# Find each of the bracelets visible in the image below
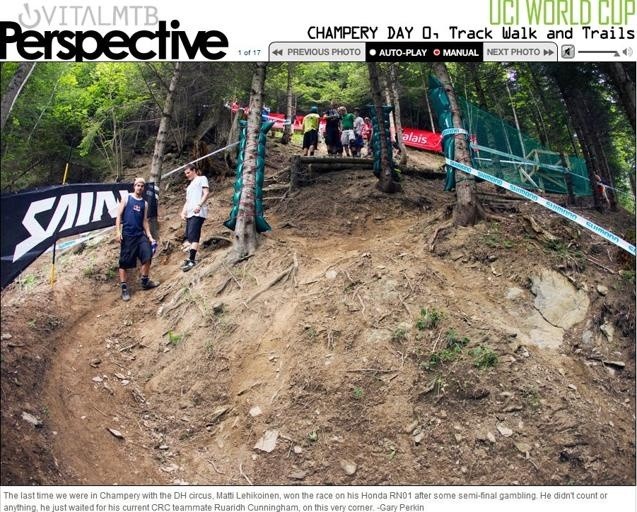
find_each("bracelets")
[198,204,202,207]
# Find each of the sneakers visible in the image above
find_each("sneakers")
[121,283,131,301]
[140,278,160,289]
[181,258,195,272]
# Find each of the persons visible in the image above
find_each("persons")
[232,101,296,135]
[115,178,159,301]
[593,169,609,204]
[301,105,320,156]
[180,164,209,271]
[322,106,374,158]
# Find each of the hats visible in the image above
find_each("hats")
[133,178,146,186]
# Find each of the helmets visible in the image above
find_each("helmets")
[309,106,318,112]
